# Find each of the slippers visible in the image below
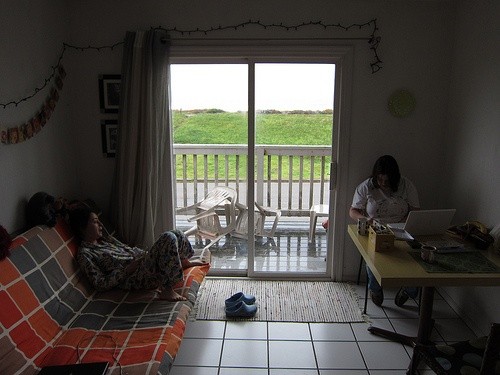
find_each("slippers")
[225,291,255,306]
[226,301,257,317]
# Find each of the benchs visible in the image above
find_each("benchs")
[0,210,211,375]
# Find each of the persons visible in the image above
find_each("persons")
[67,210,209,301]
[350,155,421,307]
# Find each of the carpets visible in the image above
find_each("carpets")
[190,279,367,323]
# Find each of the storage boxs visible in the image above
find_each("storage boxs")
[368,225,395,253]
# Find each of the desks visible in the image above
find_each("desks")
[347,224,500,375]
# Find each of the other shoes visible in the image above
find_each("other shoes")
[394,287,409,305]
[370,289,383,306]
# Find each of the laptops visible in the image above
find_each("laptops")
[387,209,456,236]
[37,362,110,375]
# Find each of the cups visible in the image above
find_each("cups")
[358,217,370,235]
[421,245,438,263]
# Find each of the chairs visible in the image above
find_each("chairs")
[175,184,281,249]
[410,323,500,375]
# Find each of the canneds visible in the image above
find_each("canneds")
[357,217,368,235]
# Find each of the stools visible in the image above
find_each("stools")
[307,204,329,243]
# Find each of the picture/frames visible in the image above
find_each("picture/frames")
[100,119,120,158]
[98,74,123,113]
